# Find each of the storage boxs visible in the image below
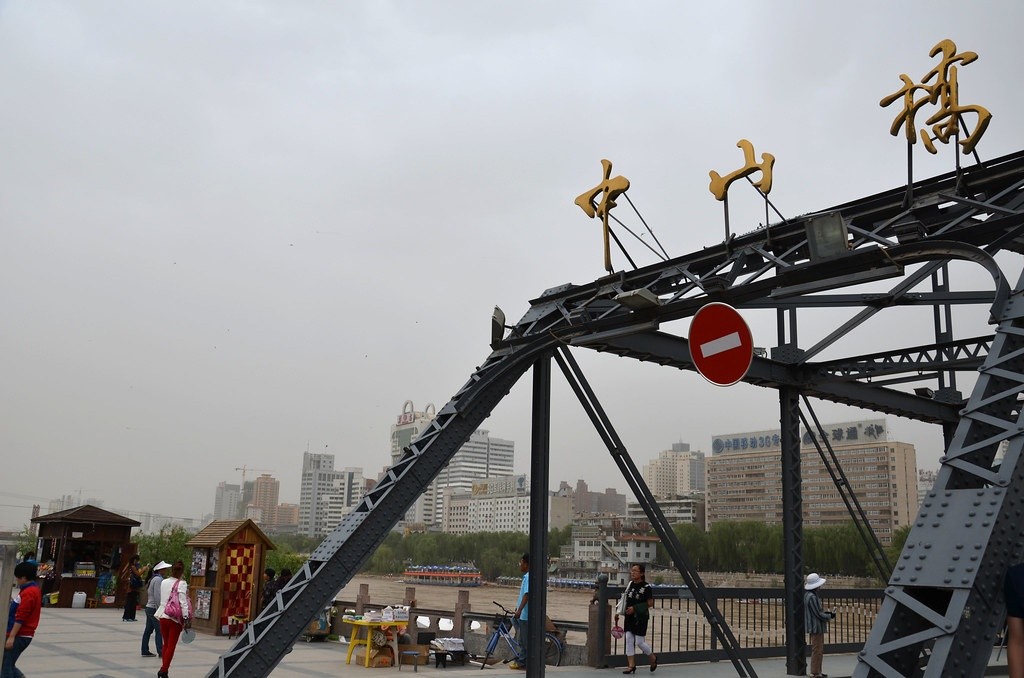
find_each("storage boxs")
[100,595,115,607]
[355,605,465,668]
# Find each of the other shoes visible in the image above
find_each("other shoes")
[814,673,828,678]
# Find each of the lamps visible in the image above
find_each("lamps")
[570,316,661,346]
[492,305,516,348]
[767,246,905,298]
[569,307,593,325]
[804,212,848,261]
[753,347,768,358]
[700,276,730,295]
[612,289,662,311]
[914,388,933,399]
[891,220,929,243]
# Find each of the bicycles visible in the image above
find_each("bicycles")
[481,600,562,670]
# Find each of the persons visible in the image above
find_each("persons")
[623,565,657,675]
[0,552,40,678]
[141,561,172,658]
[153,561,189,678]
[804,573,835,678]
[122,554,149,621]
[510,554,529,669]
[260,569,292,613]
[1005,564,1024,678]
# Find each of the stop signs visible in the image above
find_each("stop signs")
[688,301,754,387]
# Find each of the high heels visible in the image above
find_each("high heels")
[650,656,658,671]
[158,671,169,678]
[130,618,137,621]
[122,617,130,621]
[623,666,636,674]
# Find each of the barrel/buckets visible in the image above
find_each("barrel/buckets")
[72,592,86,608]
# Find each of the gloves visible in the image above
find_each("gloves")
[831,612,836,618]
[824,611,830,614]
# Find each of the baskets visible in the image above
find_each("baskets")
[492,613,512,634]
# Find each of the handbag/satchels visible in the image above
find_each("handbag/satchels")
[131,572,144,589]
[165,580,192,629]
[616,581,633,614]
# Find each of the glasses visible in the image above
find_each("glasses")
[632,569,640,572]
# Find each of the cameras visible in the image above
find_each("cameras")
[147,564,149,567]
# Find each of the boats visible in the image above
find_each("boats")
[402,561,481,587]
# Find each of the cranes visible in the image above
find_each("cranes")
[236,465,272,487]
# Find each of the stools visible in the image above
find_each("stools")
[229,615,249,640]
[431,648,467,668]
[399,651,420,672]
[86,598,98,607]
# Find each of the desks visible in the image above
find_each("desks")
[342,618,408,668]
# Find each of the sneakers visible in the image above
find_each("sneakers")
[509,661,523,670]
[159,653,162,656]
[142,651,155,656]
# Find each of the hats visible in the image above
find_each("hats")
[804,573,826,590]
[153,561,172,571]
